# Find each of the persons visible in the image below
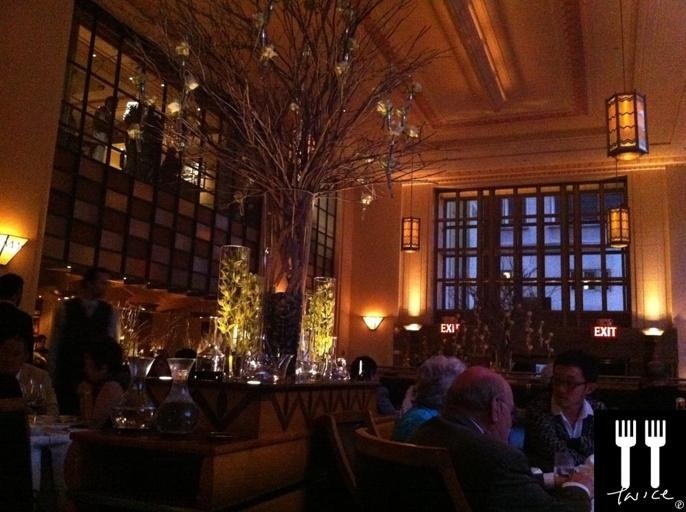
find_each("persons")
[88,96,182,177]
[350,355,608,511]
[2,267,196,512]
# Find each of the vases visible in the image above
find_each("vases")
[151,358,203,436]
[112,354,157,433]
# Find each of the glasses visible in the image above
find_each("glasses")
[549,373,588,390]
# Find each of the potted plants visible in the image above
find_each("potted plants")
[95,0,456,385]
[215,244,338,378]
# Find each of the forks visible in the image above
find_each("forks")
[613,418,669,490]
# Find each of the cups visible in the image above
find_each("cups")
[209,350,351,384]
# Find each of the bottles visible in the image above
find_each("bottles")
[110,355,203,435]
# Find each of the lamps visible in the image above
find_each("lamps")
[603,159,633,249]
[359,315,385,331]
[601,0,649,162]
[0,234,29,266]
[643,327,665,336]
[400,79,422,253]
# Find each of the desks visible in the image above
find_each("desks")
[30,422,90,512]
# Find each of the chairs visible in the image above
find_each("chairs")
[354,427,473,512]
[320,408,382,512]
